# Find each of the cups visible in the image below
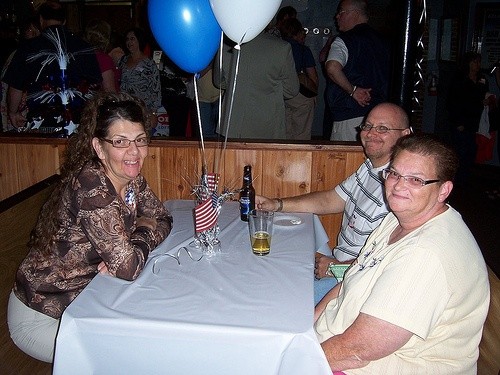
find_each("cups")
[246,208,274,256]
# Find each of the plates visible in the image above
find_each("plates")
[272,215,305,228]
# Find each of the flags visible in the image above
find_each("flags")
[194,173,221,233]
[405,0,431,134]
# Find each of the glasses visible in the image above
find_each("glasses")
[99,136,151,149]
[359,123,406,134]
[337,9,347,15]
[382,167,441,190]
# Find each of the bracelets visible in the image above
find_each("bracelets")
[349,85,357,96]
[274,198,283,212]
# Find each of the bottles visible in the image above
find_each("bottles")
[239,166,255,222]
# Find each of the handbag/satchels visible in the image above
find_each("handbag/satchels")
[296,46,317,97]
[473,127,496,164]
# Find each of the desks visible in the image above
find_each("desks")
[52,198,334,375]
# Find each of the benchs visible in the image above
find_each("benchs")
[0,172,61,375]
[475,265,500,375]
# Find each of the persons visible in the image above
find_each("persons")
[311,133,490,375]
[225,103,410,307]
[323,0,389,141]
[143,41,225,140]
[1,0,162,129]
[448,53,500,180]
[157,7,317,140]
[7,90,174,364]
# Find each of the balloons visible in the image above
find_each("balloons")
[209,0,283,49]
[148,0,223,79]
